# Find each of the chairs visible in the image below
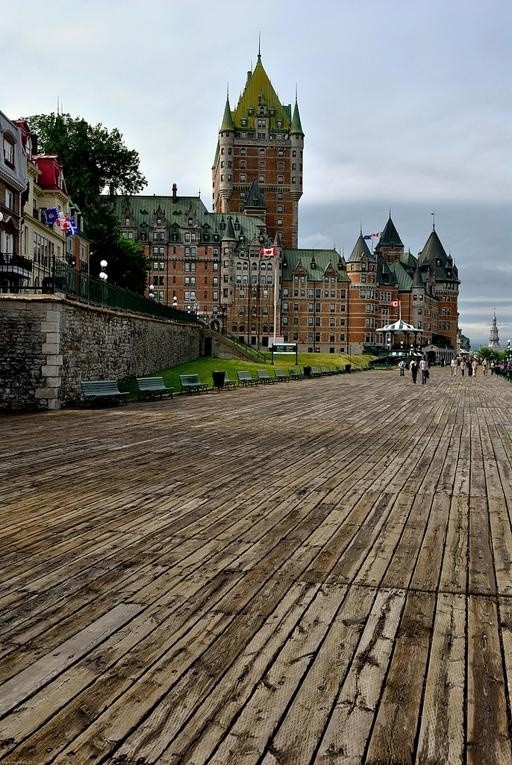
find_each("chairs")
[237,364,355,388]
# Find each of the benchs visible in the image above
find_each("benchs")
[136,374,174,398]
[179,372,209,395]
[212,369,237,390]
[81,379,130,406]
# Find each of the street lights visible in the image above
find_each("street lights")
[99,260,108,304]
[148,285,154,301]
[173,296,178,309]
[187,300,200,325]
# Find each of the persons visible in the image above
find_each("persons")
[398,359,406,376]
[436,355,512,377]
[409,356,417,384]
[419,354,429,384]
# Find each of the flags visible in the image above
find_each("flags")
[363,232,380,240]
[391,301,398,307]
[43,207,79,237]
[259,247,277,257]
